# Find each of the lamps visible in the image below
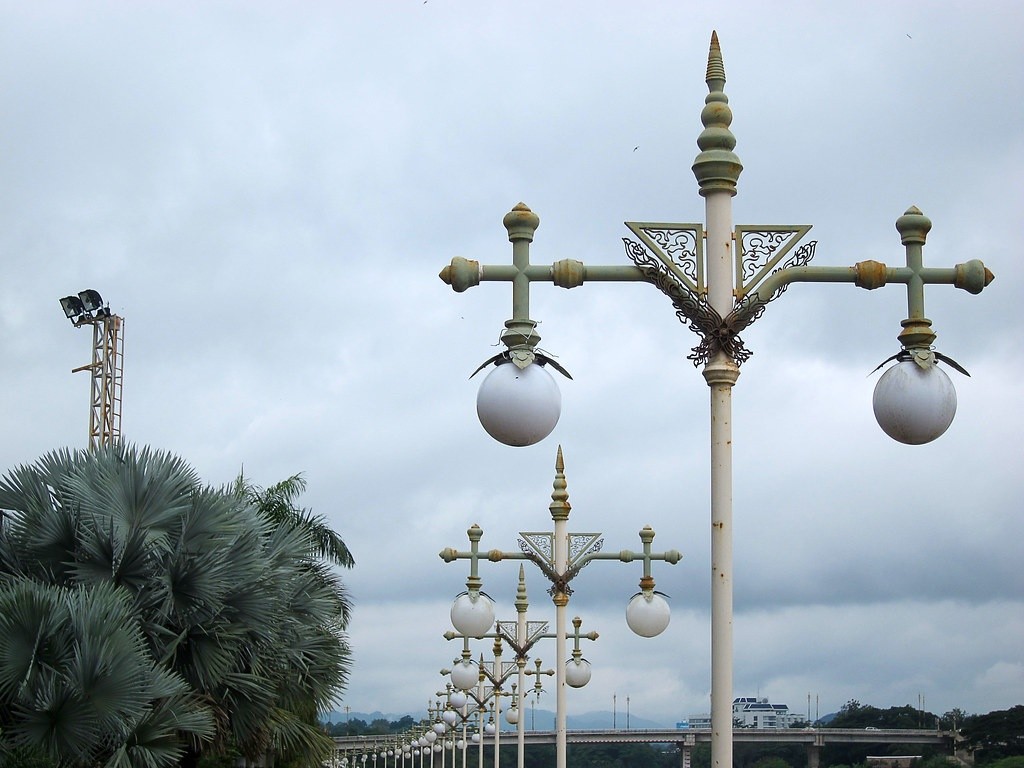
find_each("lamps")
[60,289,110,325]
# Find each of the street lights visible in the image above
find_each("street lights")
[434,446,682,768]
[441,27,993,768]
[327,563,598,768]
[58,289,122,452]
